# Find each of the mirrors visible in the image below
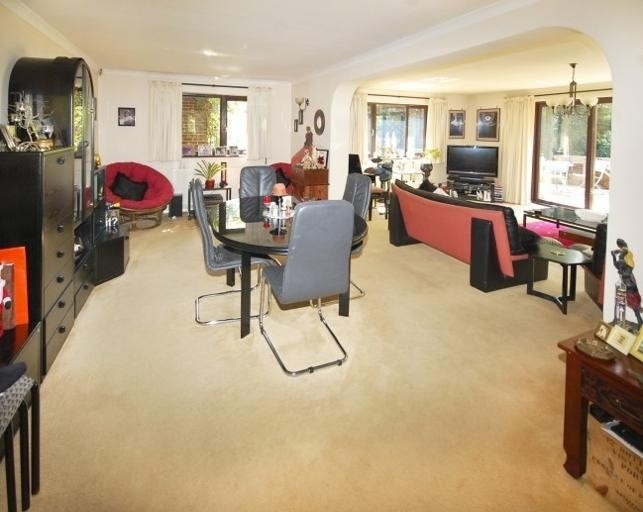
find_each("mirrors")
[72,63,94,227]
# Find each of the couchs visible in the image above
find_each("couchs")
[389,179,549,293]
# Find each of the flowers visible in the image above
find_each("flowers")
[297,146,325,170]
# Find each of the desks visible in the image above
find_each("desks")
[558,321,643,479]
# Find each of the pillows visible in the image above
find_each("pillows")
[110,172,148,200]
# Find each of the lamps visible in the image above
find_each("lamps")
[546,62,598,116]
[295,96,309,110]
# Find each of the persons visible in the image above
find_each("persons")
[123,110,133,125]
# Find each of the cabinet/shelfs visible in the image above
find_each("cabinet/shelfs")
[0,57,129,462]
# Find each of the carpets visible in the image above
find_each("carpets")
[518,221,583,248]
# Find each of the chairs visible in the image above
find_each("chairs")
[103,162,175,229]
[349,154,394,220]
[187,166,372,375]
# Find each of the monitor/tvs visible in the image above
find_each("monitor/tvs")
[447,145,499,184]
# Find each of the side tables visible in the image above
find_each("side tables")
[527,245,591,315]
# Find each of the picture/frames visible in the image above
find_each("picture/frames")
[448,110,465,139]
[476,108,500,142]
[182,145,239,156]
[118,107,135,126]
[294,110,303,132]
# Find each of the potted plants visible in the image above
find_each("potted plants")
[193,160,226,188]
